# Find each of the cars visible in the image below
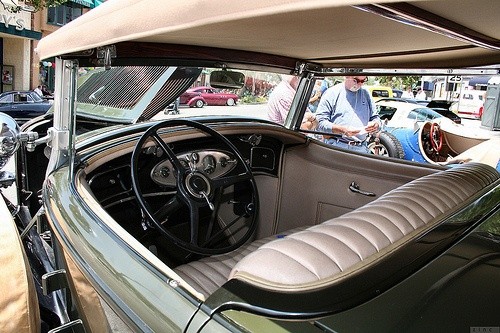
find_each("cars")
[0,90,54,125]
[363,85,500,175]
[179,86,239,108]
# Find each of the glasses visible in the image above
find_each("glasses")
[353,76,368,83]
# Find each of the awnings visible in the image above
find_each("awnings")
[92,0,106,8]
[62,0,94,9]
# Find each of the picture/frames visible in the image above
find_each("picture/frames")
[2,65,14,85]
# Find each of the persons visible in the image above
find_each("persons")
[315,75,382,154]
[266,74,316,131]
[307,80,328,113]
[415,87,427,100]
[34,85,52,97]
[401,86,415,99]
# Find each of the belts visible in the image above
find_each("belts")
[332,137,366,146]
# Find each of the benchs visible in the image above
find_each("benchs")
[171,162,500,294]
[454,140,493,162]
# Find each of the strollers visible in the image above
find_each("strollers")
[163,100,179,115]
